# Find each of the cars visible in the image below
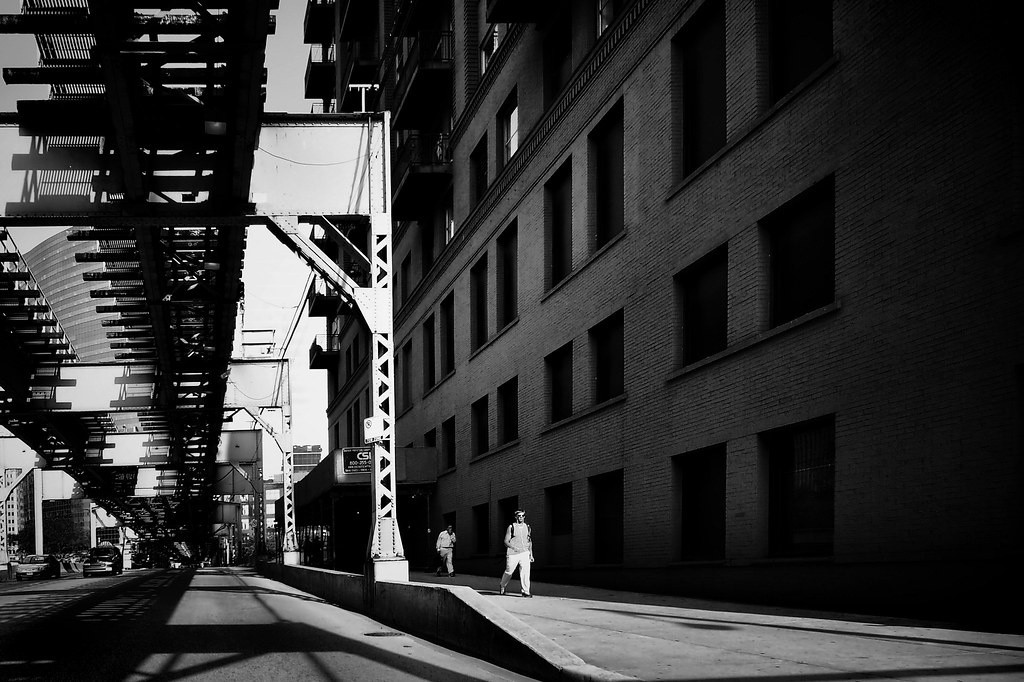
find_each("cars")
[15,554,61,581]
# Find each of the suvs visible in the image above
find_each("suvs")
[83,546,122,577]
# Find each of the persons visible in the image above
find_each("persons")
[302,535,323,566]
[436,525,456,577]
[500,510,534,597]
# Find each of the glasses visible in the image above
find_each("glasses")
[516,515,525,517]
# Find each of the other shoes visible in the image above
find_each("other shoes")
[499,584,506,595]
[448,572,455,577]
[522,593,532,598]
[437,567,442,576]
